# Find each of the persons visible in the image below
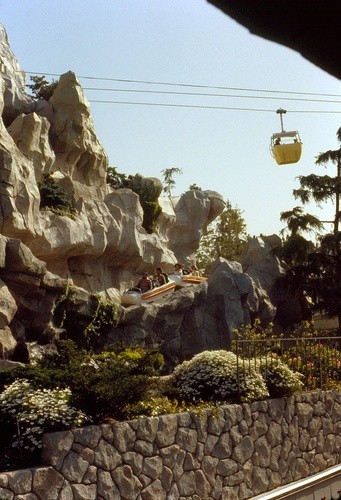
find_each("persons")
[274,140,281,146]
[149,275,155,288]
[181,265,190,274]
[292,139,298,143]
[135,272,153,293]
[188,265,199,273]
[175,263,183,276]
[161,269,170,283]
[278,138,282,145]
[152,274,161,288]
[155,267,167,286]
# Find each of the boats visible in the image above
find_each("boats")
[168,274,208,289]
[121,278,175,306]
